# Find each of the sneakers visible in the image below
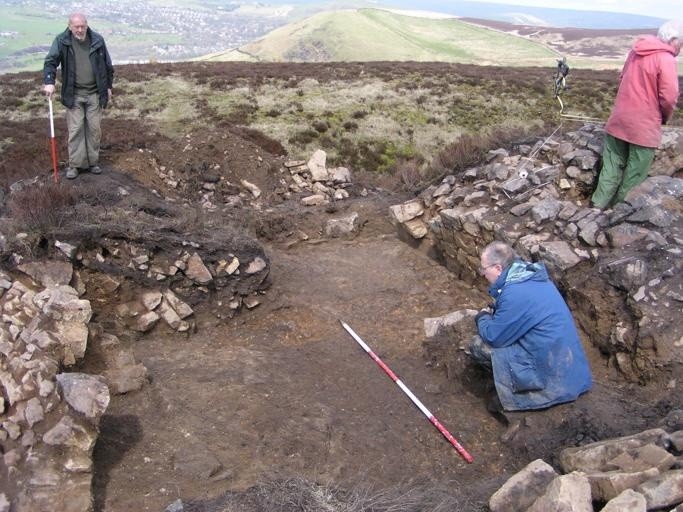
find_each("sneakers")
[87,165,102,174]
[65,167,79,179]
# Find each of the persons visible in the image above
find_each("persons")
[553,57,568,87]
[41,11,114,180]
[472,240,591,410]
[588,19,682,209]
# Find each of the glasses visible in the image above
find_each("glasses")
[479,262,500,276]
